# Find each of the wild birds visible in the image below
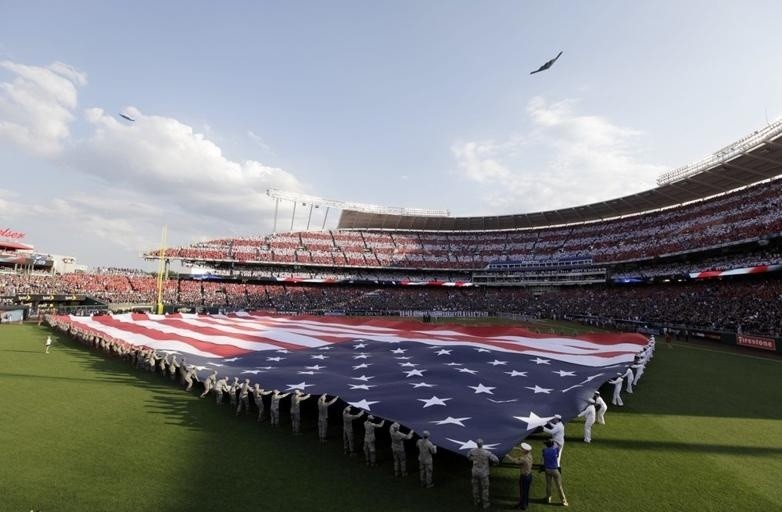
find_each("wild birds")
[529,51,563,74]
[119,113,135,121]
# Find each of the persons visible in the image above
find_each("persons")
[45,335,52,354]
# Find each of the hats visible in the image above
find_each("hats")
[520,442,532,452]
[555,365,630,419]
[272,388,484,445]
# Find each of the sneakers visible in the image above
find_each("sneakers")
[543,496,569,506]
